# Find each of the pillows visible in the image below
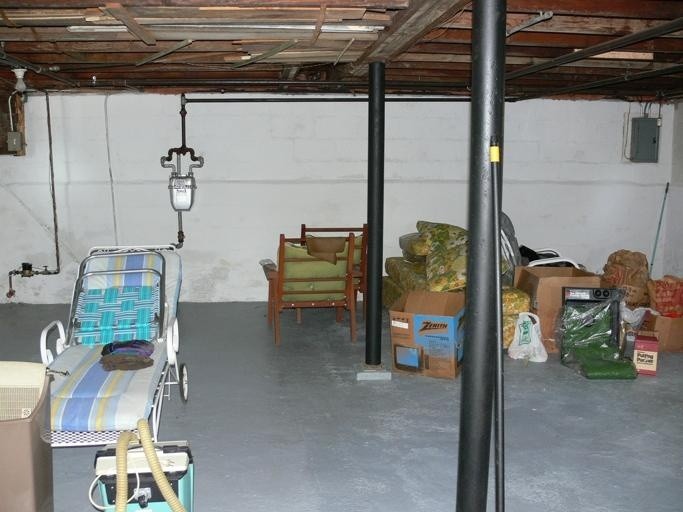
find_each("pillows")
[383,219,530,351]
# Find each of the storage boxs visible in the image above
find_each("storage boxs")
[512,265,683,358]
[388,286,469,379]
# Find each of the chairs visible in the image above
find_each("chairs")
[38,243,191,452]
[257,223,370,347]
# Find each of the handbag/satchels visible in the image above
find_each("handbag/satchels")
[507,310,548,364]
